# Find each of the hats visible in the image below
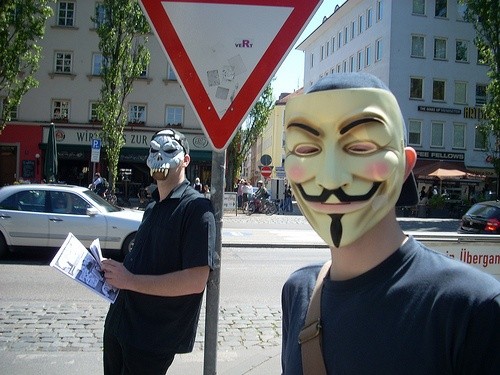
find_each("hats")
[305,71,420,211]
[150,129,189,153]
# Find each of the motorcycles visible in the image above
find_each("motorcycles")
[243,194,277,216]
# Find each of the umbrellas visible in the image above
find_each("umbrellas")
[417,165,487,196]
[45,122,58,176]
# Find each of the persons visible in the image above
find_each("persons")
[38,171,293,214]
[282,72,500,375]
[101,128,217,375]
[419,185,438,200]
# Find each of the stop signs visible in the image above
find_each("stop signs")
[261,167,272,178]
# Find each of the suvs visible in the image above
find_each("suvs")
[0,184,145,262]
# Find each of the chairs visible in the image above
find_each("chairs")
[137,193,149,210]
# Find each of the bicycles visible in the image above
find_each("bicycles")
[89,186,116,206]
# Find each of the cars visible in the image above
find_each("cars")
[459,201,500,235]
